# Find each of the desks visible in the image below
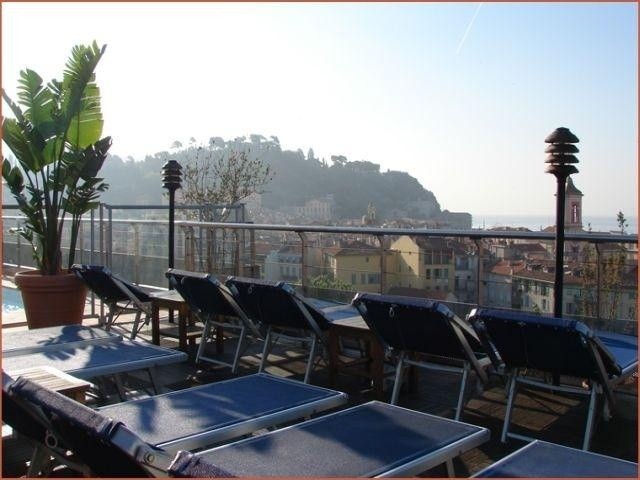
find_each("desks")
[329,315,393,402]
[147,290,205,351]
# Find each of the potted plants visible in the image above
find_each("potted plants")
[0,39,114,328]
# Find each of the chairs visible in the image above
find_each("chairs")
[468,307,640,451]
[11,372,350,479]
[352,292,510,421]
[171,401,491,478]
[164,267,361,384]
[69,262,153,340]
[470,439,638,477]
[0,324,189,455]
